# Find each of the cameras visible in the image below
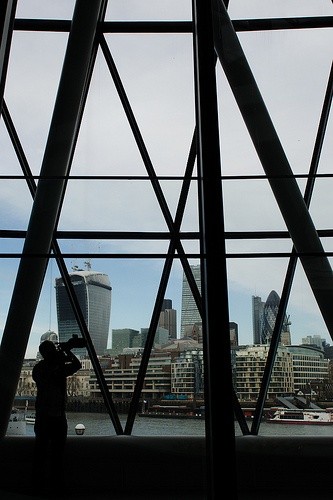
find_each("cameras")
[59,334,87,352]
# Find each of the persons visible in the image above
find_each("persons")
[32,340,82,491]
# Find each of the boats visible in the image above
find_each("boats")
[138,404,267,421]
[268,408,333,425]
[25,412,35,424]
